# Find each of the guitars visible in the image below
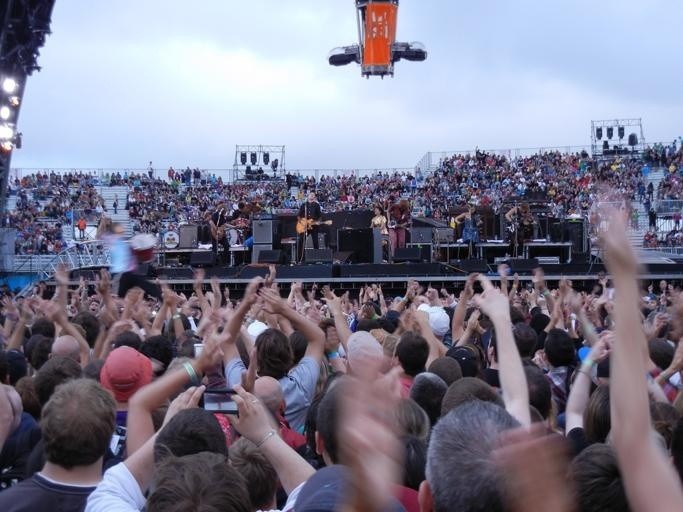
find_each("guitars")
[211,224,248,242]
[296,218,332,234]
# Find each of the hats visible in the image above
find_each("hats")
[417,303,451,337]
[98,345,154,402]
[247,321,268,343]
[445,346,476,371]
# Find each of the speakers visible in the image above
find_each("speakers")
[393,248,422,263]
[190,250,216,268]
[596,126,624,140]
[451,257,540,274]
[178,224,199,248]
[410,223,432,243]
[303,232,333,264]
[548,221,588,255]
[251,243,282,264]
[252,220,282,245]
[241,153,269,165]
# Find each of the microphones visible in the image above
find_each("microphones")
[304,194,309,197]
[218,208,224,214]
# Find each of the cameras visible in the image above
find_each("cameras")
[198,389,240,414]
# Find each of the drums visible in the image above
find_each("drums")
[163,232,180,249]
[131,235,157,264]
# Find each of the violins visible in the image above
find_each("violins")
[470,213,483,224]
